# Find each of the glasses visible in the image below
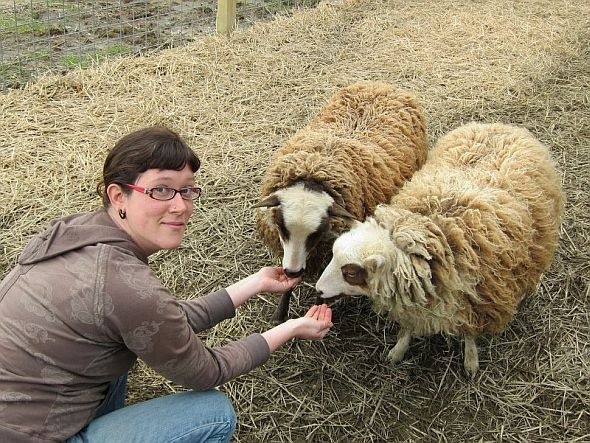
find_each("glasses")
[121,182,201,200]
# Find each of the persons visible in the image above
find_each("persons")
[0,126,335,441]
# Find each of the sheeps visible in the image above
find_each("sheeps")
[247,78,429,340]
[313,120,568,378]
